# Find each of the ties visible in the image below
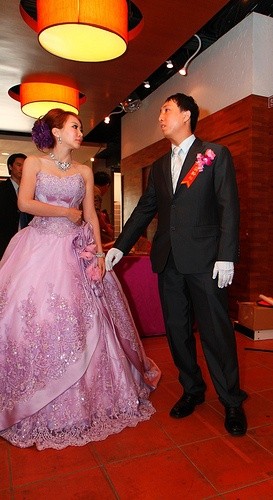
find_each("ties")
[17,187,28,230]
[173,147,182,194]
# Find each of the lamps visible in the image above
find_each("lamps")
[178,34,202,76]
[18,0,145,64]
[8,83,87,119]
[104,98,142,124]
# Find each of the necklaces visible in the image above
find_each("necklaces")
[48,151,73,172]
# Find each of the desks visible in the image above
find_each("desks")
[110,255,198,338]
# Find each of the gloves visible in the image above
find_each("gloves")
[212,261,234,288]
[105,248,123,271]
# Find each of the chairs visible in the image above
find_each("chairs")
[103,237,151,256]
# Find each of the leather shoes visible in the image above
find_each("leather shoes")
[169,393,205,418]
[224,406,248,435]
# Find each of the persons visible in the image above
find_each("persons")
[0,108,163,451]
[88,171,111,247]
[0,152,36,267]
[102,92,248,435]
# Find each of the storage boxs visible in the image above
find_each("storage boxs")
[237,302,273,331]
